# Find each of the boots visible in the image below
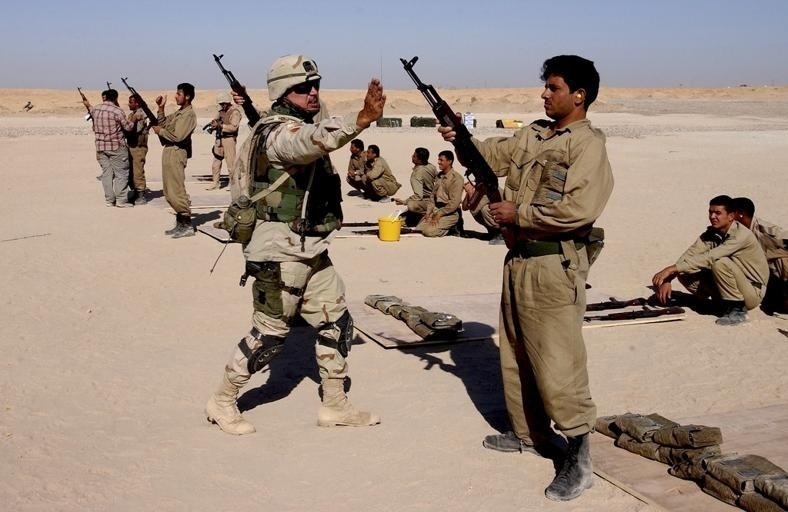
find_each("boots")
[226,182,233,191]
[172,215,195,238]
[483,429,551,456]
[165,214,180,235]
[544,434,596,504]
[488,233,506,245]
[205,370,257,437]
[135,191,149,205]
[205,181,220,191]
[316,378,383,428]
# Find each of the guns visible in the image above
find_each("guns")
[213,117,223,148]
[75,86,97,133]
[210,52,262,127]
[397,56,528,250]
[299,158,323,253]
[104,80,119,107]
[119,76,167,147]
[341,221,422,234]
[581,296,686,323]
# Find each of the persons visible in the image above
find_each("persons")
[152,83,197,238]
[396,147,506,245]
[652,195,787,324]
[204,52,387,436]
[436,54,616,502]
[85,88,149,209]
[347,137,402,203]
[205,95,242,192]
[24,101,34,112]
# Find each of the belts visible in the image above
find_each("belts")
[512,238,585,257]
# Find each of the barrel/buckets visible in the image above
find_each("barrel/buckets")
[378,209,403,242]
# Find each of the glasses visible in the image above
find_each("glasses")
[285,80,323,98]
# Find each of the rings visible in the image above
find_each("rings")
[494,215,496,220]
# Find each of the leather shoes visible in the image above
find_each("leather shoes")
[716,306,749,326]
[117,203,134,208]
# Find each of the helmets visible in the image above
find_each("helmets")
[266,53,322,102]
[217,91,232,105]
